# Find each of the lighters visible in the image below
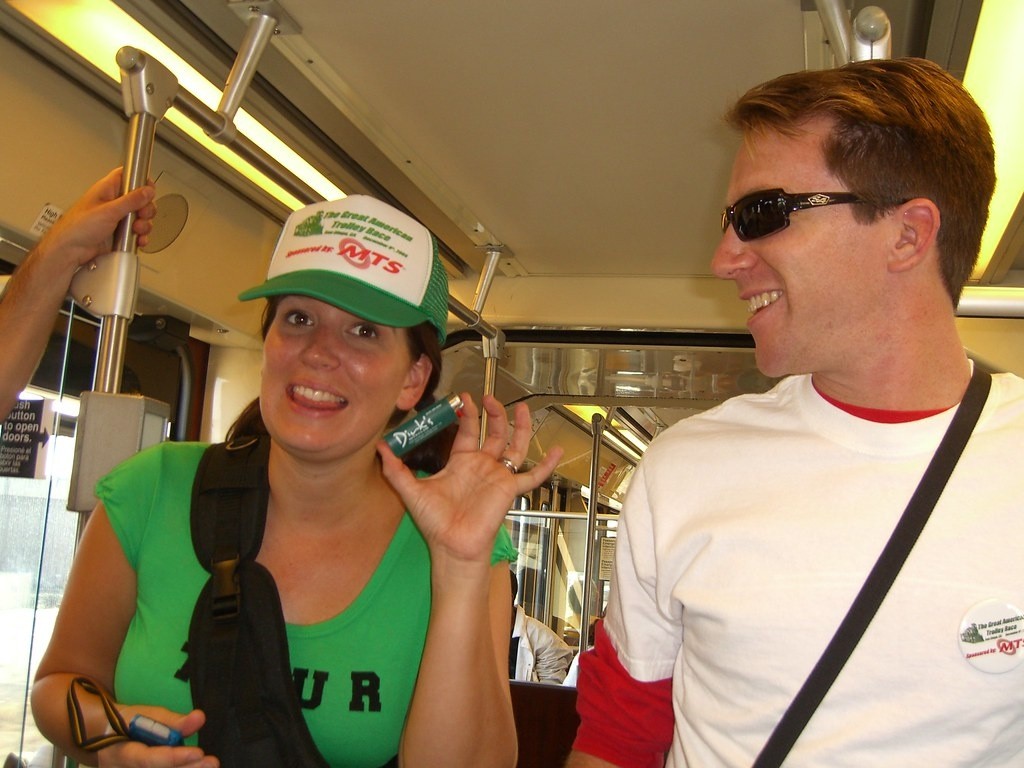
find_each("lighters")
[385,392,465,458]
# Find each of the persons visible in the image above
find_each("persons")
[565,55,1024,768]
[29,193,565,768]
[506,567,612,687]
[0,164,157,425]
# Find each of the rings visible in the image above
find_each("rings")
[501,456,518,474]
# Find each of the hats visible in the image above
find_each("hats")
[238,193,447,348]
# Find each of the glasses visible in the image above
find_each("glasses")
[722,188,867,242]
